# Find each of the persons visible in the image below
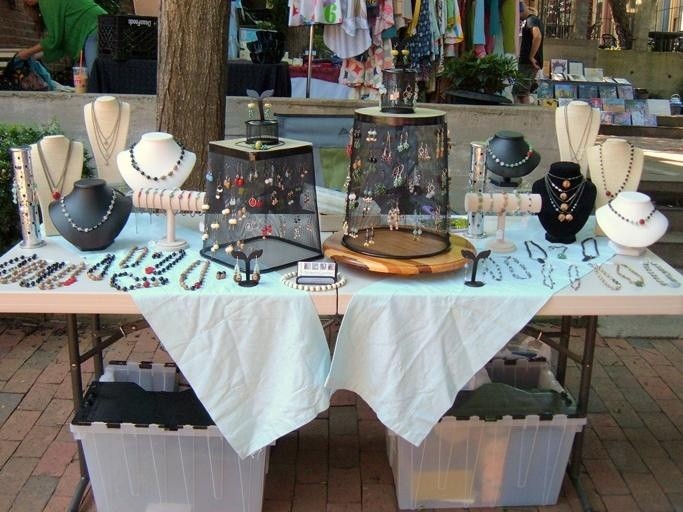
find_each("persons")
[17,0,110,78]
[511,7,543,104]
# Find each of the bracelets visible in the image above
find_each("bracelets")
[281,270,350,292]
[475,190,532,216]
[134,185,205,217]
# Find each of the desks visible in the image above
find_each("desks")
[0,213,683,512]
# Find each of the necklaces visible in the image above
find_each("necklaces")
[129,141,184,181]
[1,245,211,290]
[486,138,533,167]
[478,233,679,292]
[545,172,587,223]
[564,106,593,165]
[60,189,116,233]
[91,100,121,166]
[608,199,656,225]
[37,139,73,200]
[600,141,634,197]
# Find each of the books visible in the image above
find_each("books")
[536,58,657,127]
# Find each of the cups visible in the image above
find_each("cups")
[72,67,89,93]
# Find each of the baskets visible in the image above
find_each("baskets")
[97,15,158,61]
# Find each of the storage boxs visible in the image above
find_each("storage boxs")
[70,359,276,512]
[385,357,587,511]
